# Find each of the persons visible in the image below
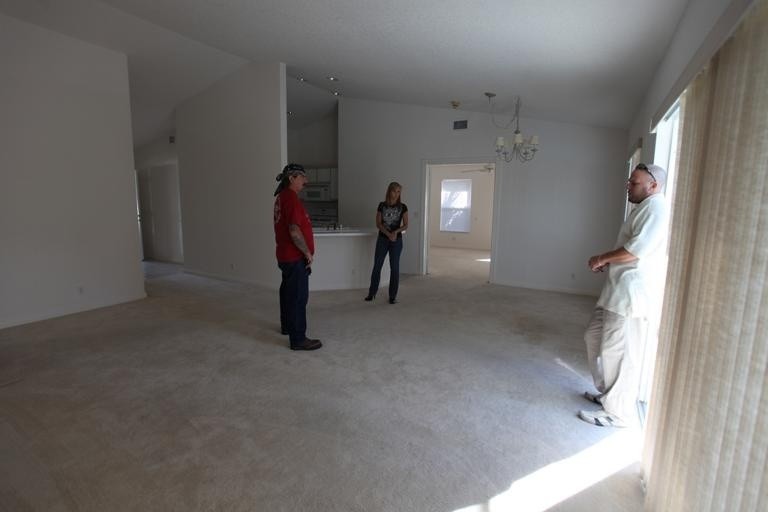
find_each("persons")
[579,163,669,429]
[273,163,322,351]
[365,181,409,305]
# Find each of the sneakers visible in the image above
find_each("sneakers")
[281,330,322,351]
[365,295,396,304]
[578,391,630,427]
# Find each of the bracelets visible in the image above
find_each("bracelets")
[597,254,602,266]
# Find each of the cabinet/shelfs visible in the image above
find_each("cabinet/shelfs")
[297,168,338,202]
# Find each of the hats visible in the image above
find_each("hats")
[636,163,656,182]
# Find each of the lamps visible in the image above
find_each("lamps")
[494,97,539,163]
